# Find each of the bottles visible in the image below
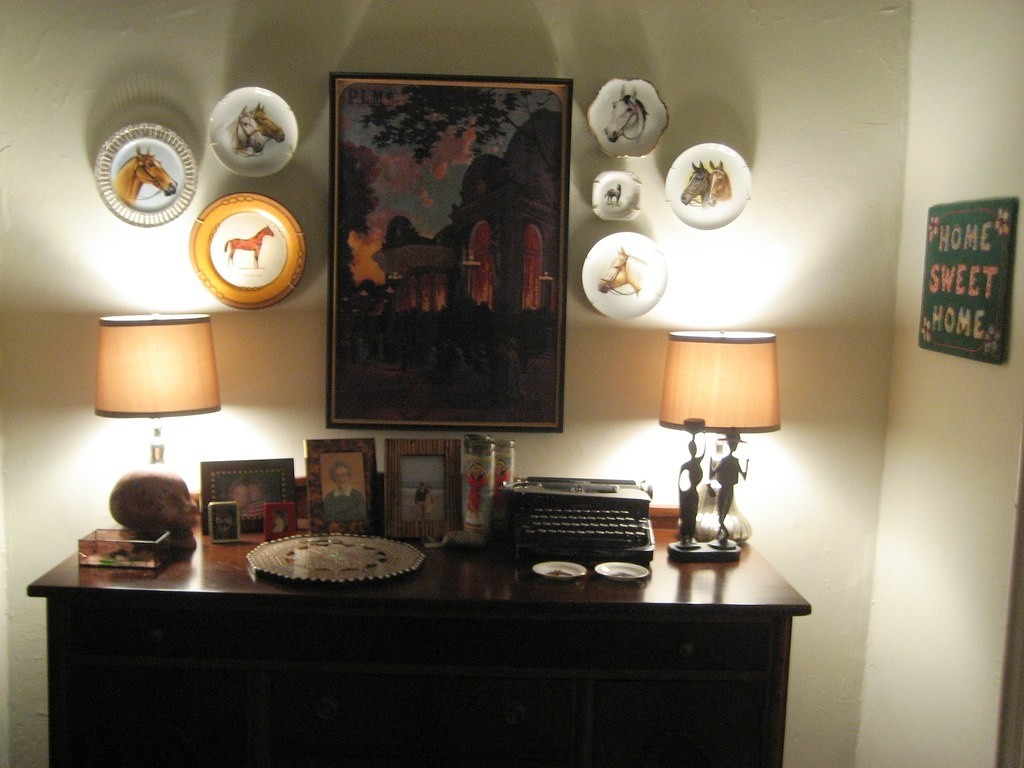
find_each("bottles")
[462,432,515,533]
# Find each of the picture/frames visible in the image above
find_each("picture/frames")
[207,501,241,543]
[381,439,461,539]
[263,501,296,542]
[303,438,378,536]
[326,72,574,434]
[201,458,295,535]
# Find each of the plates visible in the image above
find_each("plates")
[586,77,669,158]
[93,122,197,227]
[595,562,650,581]
[665,142,752,231]
[208,86,300,178]
[189,192,305,310]
[532,561,587,581]
[592,170,643,221]
[582,231,669,319]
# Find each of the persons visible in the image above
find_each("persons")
[228,479,269,520]
[414,483,434,519]
[324,461,366,520]
[677,440,708,542]
[708,432,750,541]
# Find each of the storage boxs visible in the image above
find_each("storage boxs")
[79,529,171,569]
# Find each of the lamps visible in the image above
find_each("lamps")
[93,313,222,472]
[661,330,779,564]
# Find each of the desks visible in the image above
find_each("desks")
[27,506,813,768]
